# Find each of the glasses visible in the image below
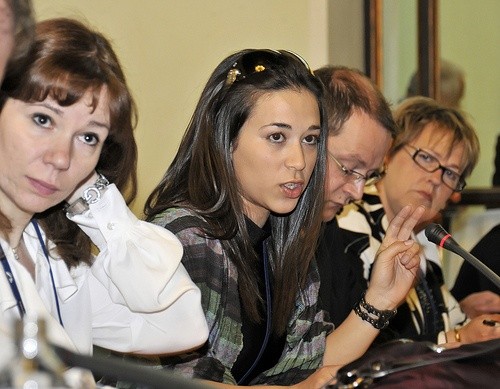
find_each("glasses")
[407,144,467,193]
[329,150,382,187]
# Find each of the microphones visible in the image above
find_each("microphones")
[424,222,500,289]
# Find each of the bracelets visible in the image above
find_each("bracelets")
[353,290,397,330]
[454,328,461,343]
[62,173,110,217]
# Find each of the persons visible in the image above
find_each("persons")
[336,96,500,352]
[0,17,210,389]
[450,224,500,303]
[0,0,36,86]
[302,64,398,330]
[95,49,425,389]
[397,58,465,233]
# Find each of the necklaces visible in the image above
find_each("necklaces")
[0,218,65,327]
[9,239,21,260]
